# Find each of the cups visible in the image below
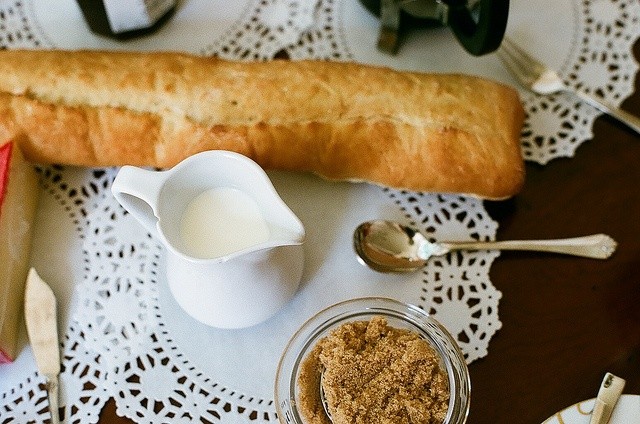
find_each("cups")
[109,151,308,330]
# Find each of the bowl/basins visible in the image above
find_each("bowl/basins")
[274,295,475,424]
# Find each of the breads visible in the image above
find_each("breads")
[0,49,526,199]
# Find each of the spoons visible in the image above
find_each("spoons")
[352,218,617,275]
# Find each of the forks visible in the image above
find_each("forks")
[495,35,640,136]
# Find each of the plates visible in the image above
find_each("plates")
[543,379,636,424]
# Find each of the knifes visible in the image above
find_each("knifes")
[22,266,65,421]
